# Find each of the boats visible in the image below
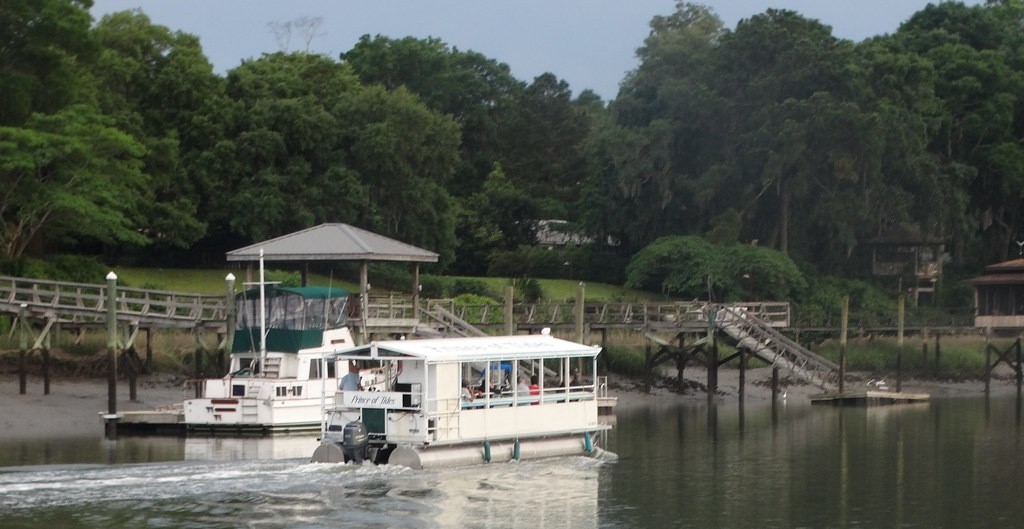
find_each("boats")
[96,281,360,438]
[311,327,614,472]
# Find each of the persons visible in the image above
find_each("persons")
[557,372,582,403]
[510,376,531,406]
[528,376,539,406]
[477,379,494,409]
[462,379,474,410]
[339,364,361,391]
